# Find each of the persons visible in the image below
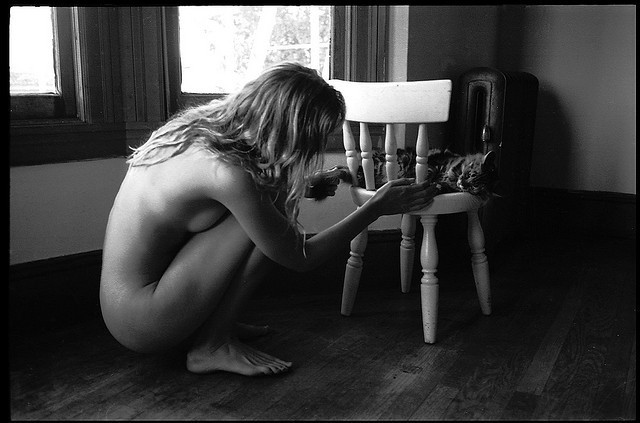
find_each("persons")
[99,63,441,376]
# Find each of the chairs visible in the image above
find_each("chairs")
[325,78,492,344]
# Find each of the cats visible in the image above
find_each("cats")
[310,149,502,203]
